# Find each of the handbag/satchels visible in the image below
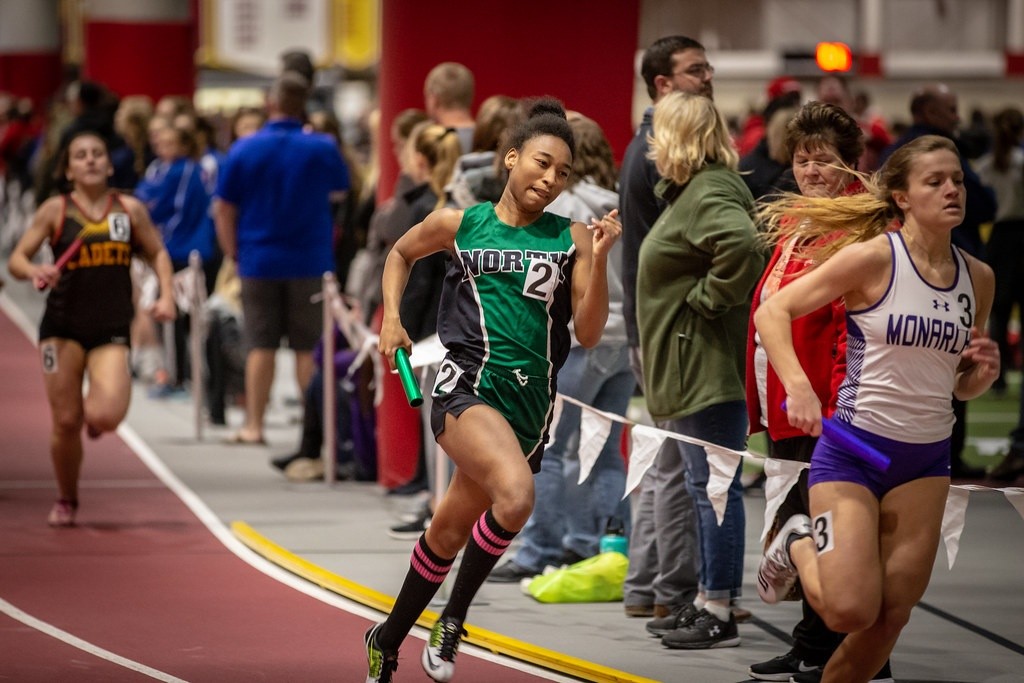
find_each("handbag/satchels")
[520,551,630,604]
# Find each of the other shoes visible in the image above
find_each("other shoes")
[653,603,677,618]
[46,497,77,528]
[86,425,102,439]
[624,604,653,617]
[273,452,306,470]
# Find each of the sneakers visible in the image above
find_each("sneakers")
[789,657,894,682]
[486,559,537,584]
[363,622,406,683]
[757,515,812,605]
[421,617,469,683]
[645,602,698,637]
[388,500,435,540]
[749,652,824,681]
[660,609,742,648]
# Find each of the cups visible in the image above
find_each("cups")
[600,534,628,556]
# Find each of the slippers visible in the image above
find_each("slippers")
[224,428,265,445]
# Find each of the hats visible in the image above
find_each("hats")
[768,77,800,103]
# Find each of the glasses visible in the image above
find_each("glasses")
[666,66,715,78]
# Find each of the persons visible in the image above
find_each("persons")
[619,35,713,619]
[746,99,902,683]
[731,71,1024,485]
[364,97,623,683]
[112,49,625,582]
[753,136,1001,683]
[9,121,176,525]
[636,91,773,650]
[0,74,137,207]
